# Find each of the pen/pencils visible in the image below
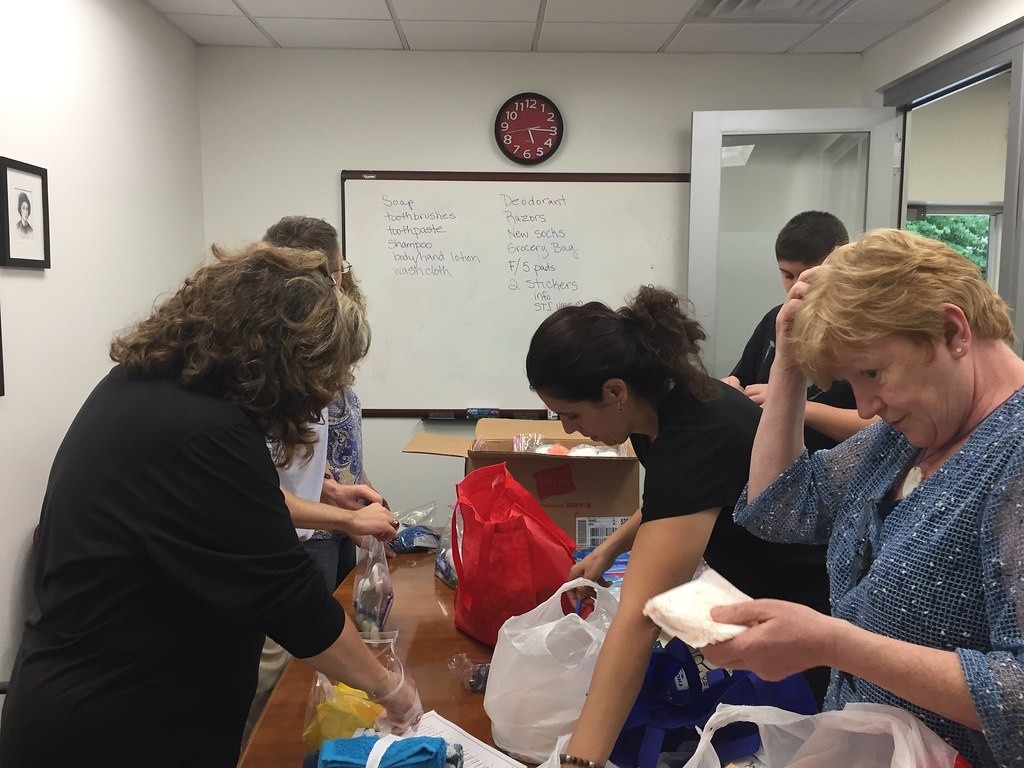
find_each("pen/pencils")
[574,599,583,614]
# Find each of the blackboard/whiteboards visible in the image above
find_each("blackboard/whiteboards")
[340,169,691,423]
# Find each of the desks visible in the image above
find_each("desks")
[234,527,707,768]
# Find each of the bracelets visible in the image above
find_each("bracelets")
[559,754,600,768]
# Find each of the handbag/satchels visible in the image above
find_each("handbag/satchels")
[452,462,595,648]
[483,577,972,768]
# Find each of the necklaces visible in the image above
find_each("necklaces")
[902,385,1024,498]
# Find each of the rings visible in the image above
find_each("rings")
[391,518,399,529]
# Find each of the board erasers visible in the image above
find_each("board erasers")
[428,412,455,421]
[465,408,500,420]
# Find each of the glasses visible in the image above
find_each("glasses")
[325,274,336,287]
[330,260,351,274]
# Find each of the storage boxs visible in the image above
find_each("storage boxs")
[402,417,657,551]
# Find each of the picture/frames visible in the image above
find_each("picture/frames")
[0,155,52,268]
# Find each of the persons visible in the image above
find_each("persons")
[522,286,832,768]
[721,209,885,458]
[694,227,1024,768]
[0,235,424,768]
[242,213,400,749]
[14,190,33,234]
[301,373,397,589]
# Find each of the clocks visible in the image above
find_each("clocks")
[494,92,563,164]
